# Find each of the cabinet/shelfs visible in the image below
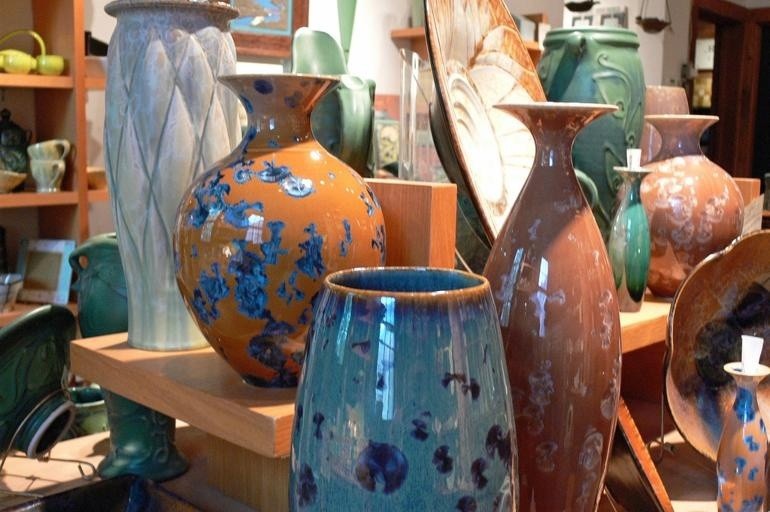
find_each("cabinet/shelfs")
[0,3,118,330]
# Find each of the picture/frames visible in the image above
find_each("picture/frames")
[223,1,310,58]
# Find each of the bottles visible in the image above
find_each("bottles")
[69,0,770,512]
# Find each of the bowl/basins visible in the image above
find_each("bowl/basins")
[37,55,64,75]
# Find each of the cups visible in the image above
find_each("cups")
[27,139,69,192]
[1,274,23,311]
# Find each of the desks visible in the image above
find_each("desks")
[70,293,674,510]
